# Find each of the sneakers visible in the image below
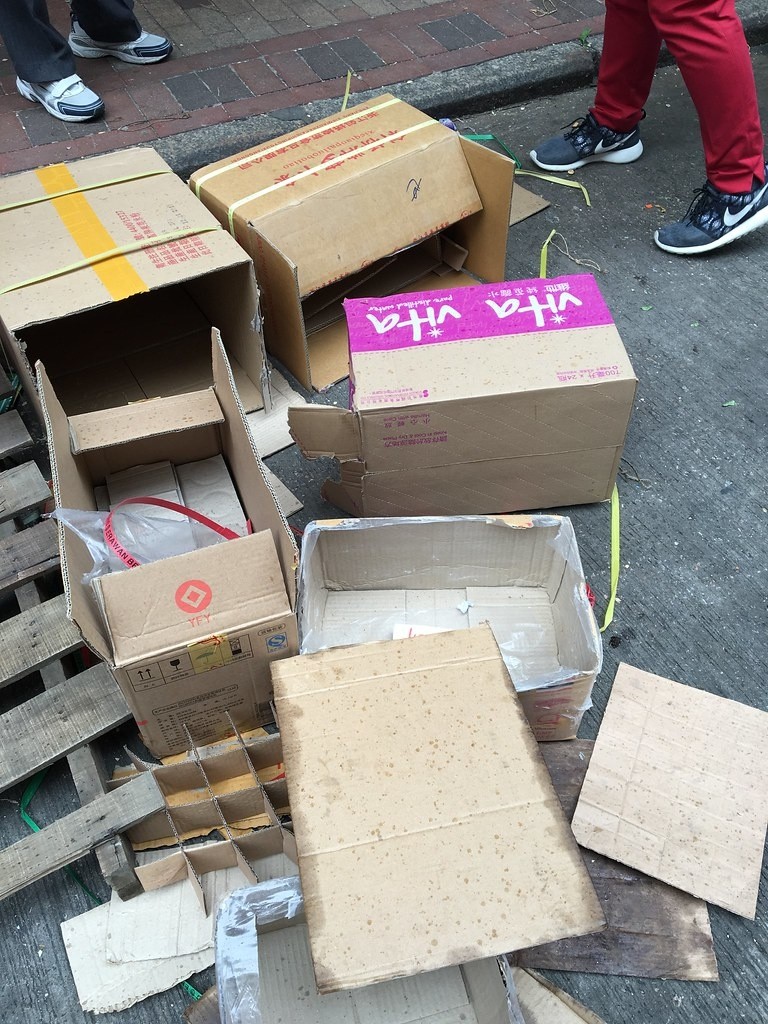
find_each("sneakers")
[654,167,768,254]
[16,72,105,122]
[68,12,174,63]
[529,110,645,172]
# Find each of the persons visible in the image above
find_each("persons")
[0,1,174,123]
[526,0,768,253]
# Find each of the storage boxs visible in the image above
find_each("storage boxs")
[0,93,604,748]
